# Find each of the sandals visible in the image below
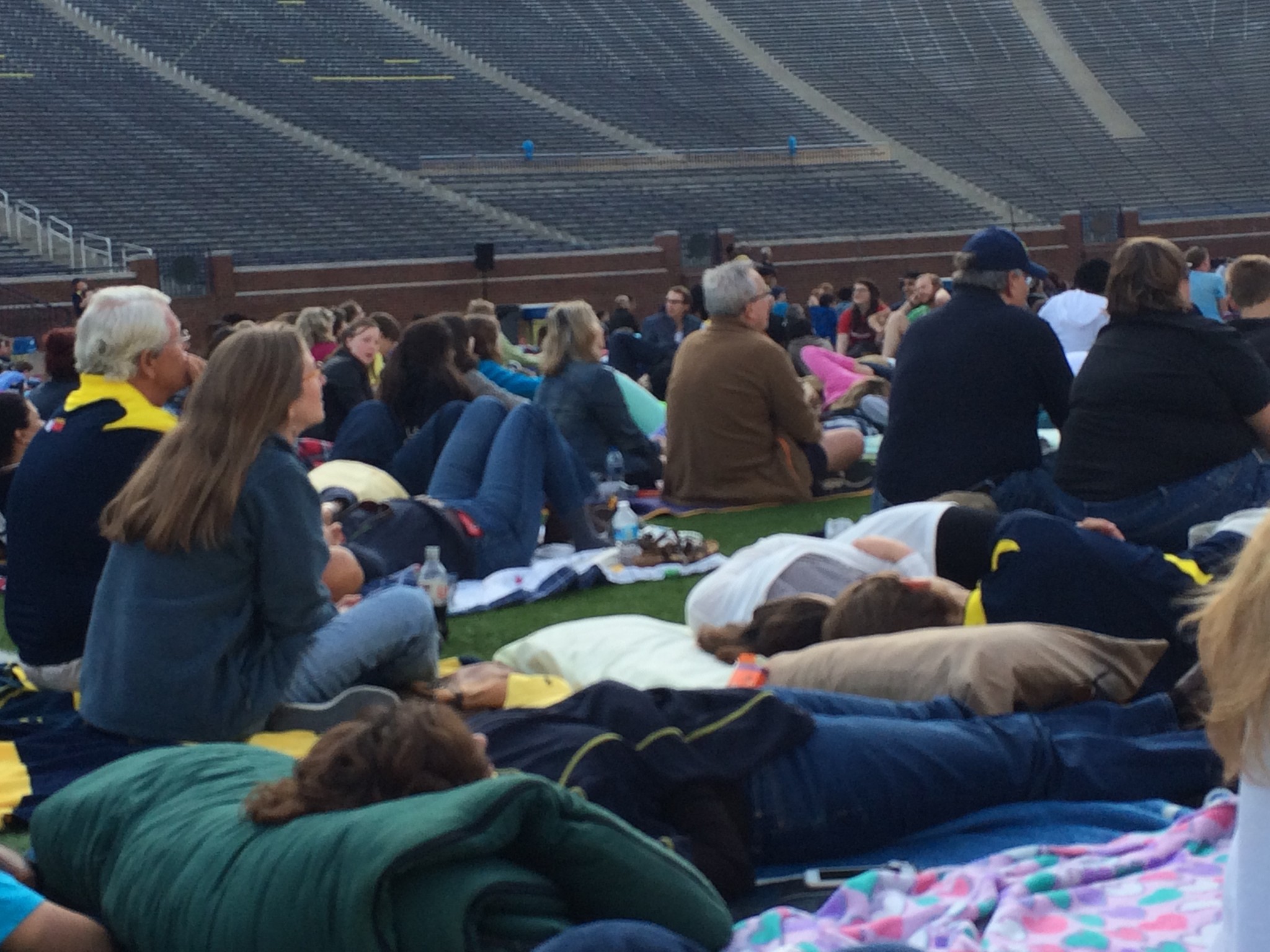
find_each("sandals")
[632,532,718,567]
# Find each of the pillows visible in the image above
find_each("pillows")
[766,618,1170,711]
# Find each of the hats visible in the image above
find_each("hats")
[953,224,1050,279]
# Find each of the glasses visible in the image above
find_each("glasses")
[751,285,781,303]
[169,328,193,352]
[1184,261,1195,274]
[300,360,327,384]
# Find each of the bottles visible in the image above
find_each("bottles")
[611,500,640,547]
[416,544,451,642]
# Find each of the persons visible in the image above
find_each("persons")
[0,219,1267,952]
